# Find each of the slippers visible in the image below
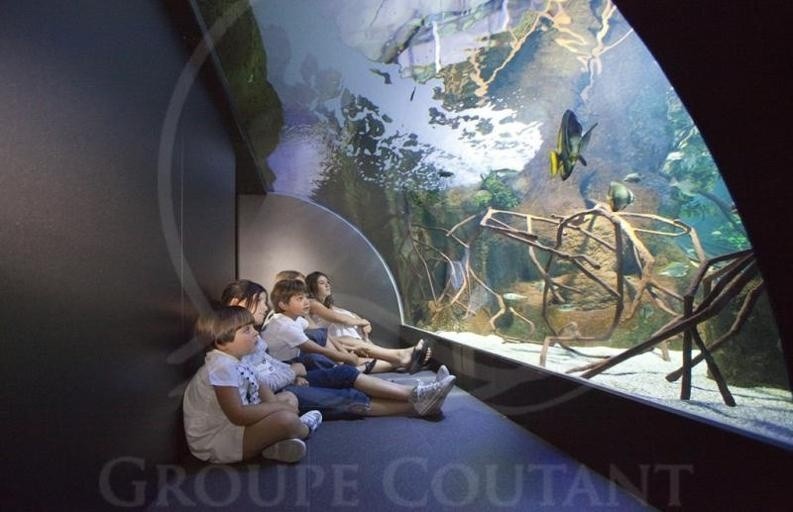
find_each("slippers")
[410,343,432,374]
[360,359,375,374]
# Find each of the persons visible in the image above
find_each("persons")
[218,278,457,421]
[257,280,378,374]
[182,305,323,464]
[305,271,375,346]
[274,270,432,373]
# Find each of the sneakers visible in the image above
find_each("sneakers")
[412,365,457,417]
[263,438,307,464]
[299,410,323,433]
